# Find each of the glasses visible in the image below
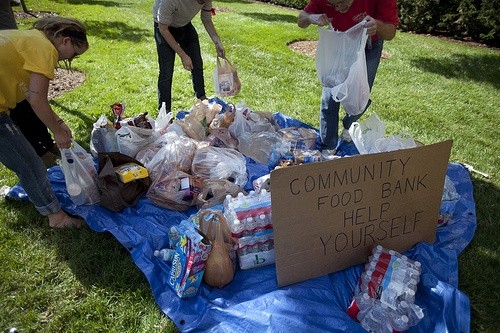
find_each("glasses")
[72,44,80,59]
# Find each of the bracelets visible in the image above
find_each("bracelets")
[57,119,63,126]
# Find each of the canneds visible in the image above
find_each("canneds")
[68,177,100,205]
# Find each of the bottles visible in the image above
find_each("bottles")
[169,225,182,248]
[224,189,275,270]
[154,249,175,264]
[348,246,424,333]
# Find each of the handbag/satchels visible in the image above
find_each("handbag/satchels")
[214,55,241,97]
[57,97,417,208]
[316,16,370,115]
[199,209,238,288]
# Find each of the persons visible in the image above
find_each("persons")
[152,0,225,122]
[298,0,399,157]
[0,16,90,230]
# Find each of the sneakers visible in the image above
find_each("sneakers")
[321,147,337,157]
[341,126,352,144]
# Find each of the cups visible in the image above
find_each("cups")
[68,185,84,205]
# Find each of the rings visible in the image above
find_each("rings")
[57,146,60,148]
[374,30,376,32]
